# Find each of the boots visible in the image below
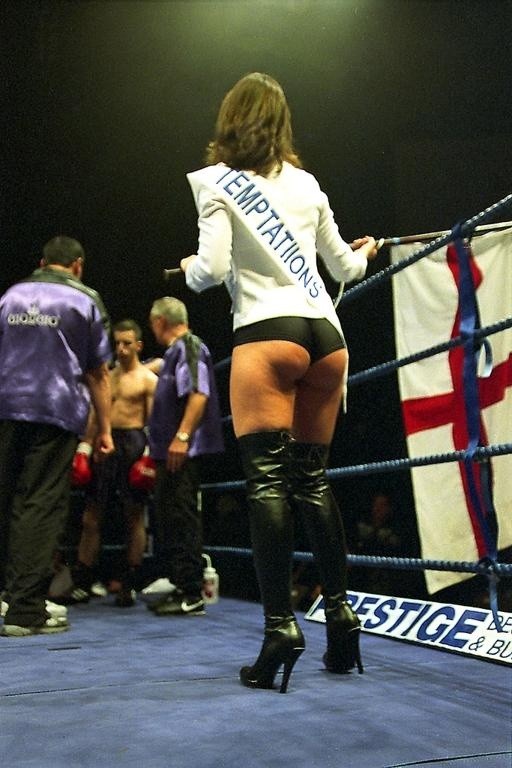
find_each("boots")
[289,443,366,675]
[237,429,306,694]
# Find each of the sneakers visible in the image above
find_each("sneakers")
[102,588,137,608]
[47,586,91,610]
[145,588,184,612]
[153,594,207,619]
[1,611,69,637]
[1,597,69,620]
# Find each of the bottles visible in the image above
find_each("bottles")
[201,554,221,604]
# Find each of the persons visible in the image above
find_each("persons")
[70,318,160,607]
[140,356,165,374]
[147,296,224,618]
[179,71,378,693]
[0,235,116,638]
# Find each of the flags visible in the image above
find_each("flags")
[387,226,512,596]
